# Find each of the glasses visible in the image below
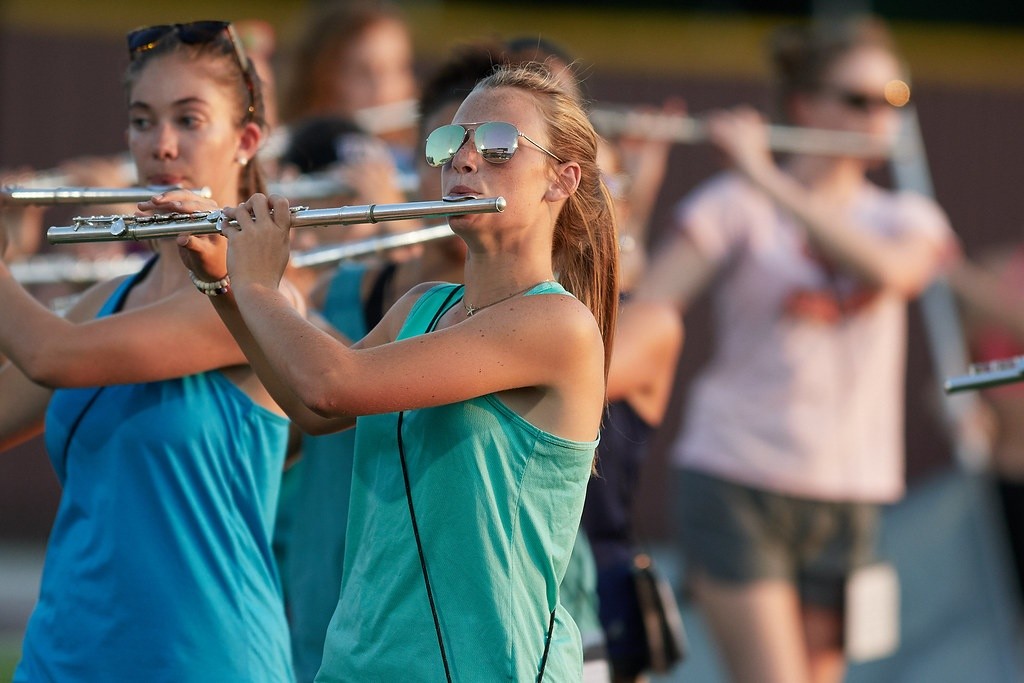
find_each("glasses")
[833,87,912,115]
[424,122,566,169]
[127,20,249,75]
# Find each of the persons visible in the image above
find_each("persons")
[133,70,619,683]
[0,11,954,683]
[0,20,306,683]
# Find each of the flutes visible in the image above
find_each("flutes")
[588,104,897,163]
[0,183,210,206]
[48,193,508,244]
[941,353,1023,398]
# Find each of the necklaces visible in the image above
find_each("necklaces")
[462,279,556,316]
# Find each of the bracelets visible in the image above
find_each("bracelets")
[189,270,231,296]
